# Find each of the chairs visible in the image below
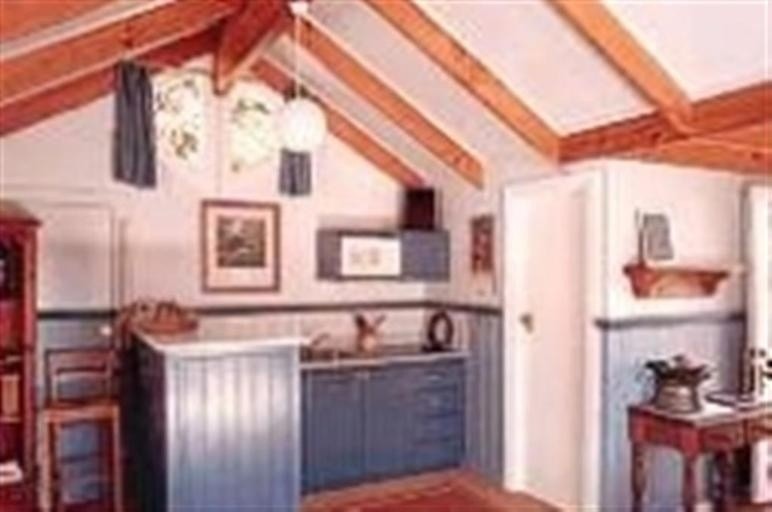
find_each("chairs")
[38,348,124,512]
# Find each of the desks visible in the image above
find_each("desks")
[628,393,772,512]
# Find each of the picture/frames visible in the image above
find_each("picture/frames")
[637,208,677,269]
[202,199,281,293]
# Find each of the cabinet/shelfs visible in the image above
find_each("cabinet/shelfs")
[300,362,467,494]
[399,227,449,281]
[0,199,39,511]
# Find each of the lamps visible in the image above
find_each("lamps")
[277,1,327,155]
